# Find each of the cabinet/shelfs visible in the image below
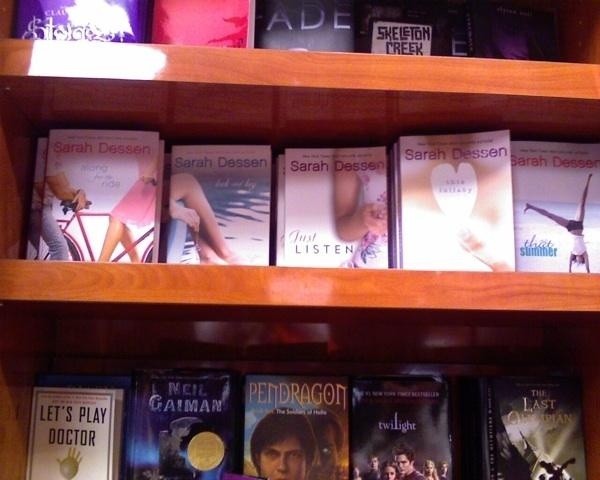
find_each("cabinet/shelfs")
[0,2,600,480]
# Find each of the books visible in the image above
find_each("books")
[30,130,600,273]
[10,1,566,63]
[24,339,587,480]
